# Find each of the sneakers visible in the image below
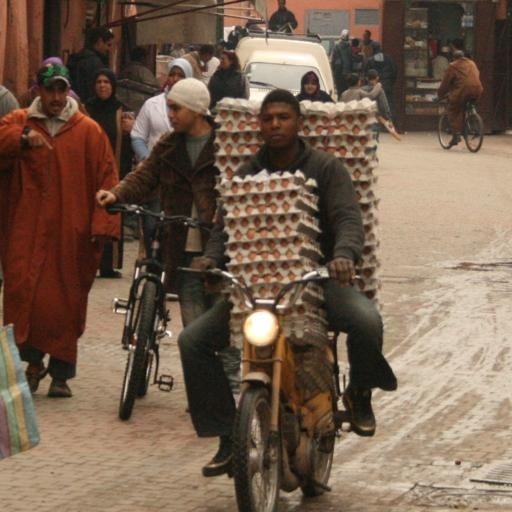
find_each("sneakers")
[48,381,72,397]
[203,448,234,477]
[343,388,375,435]
[26,364,46,392]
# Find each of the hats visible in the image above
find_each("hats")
[168,77,212,115]
[37,57,71,87]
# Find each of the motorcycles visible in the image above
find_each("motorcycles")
[178,264,363,512]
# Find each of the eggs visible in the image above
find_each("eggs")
[212,109,382,300]
[222,176,315,319]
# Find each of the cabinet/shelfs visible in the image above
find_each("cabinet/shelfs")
[403,7,449,116]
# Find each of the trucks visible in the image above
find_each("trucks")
[235,28,337,106]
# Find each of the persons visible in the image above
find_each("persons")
[1,82,24,116]
[17,0,485,147]
[1,57,120,399]
[128,58,196,299]
[96,79,242,411]
[175,88,398,478]
[83,71,133,277]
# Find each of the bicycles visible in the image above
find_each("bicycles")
[434,93,485,155]
[96,192,212,424]
